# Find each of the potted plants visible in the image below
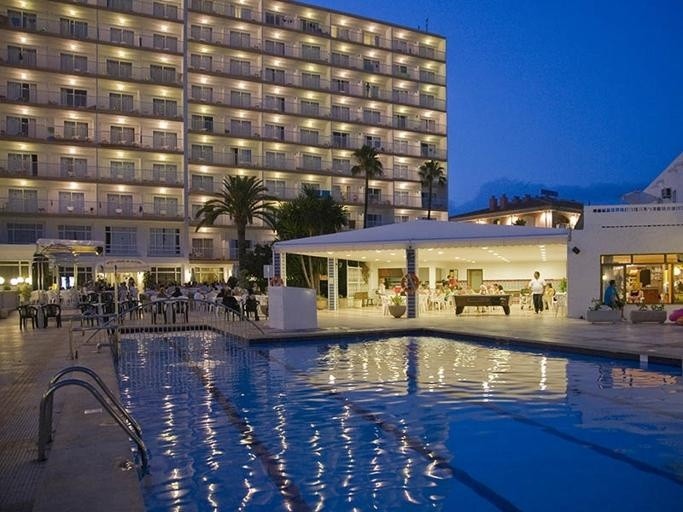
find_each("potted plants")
[587,298,625,324]
[630,300,666,323]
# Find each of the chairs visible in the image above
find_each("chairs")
[17,290,268,330]
[379,292,568,317]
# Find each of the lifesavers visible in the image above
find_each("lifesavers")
[400,273,419,293]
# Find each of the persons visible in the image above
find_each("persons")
[98,276,284,322]
[604,280,627,320]
[443,271,557,315]
